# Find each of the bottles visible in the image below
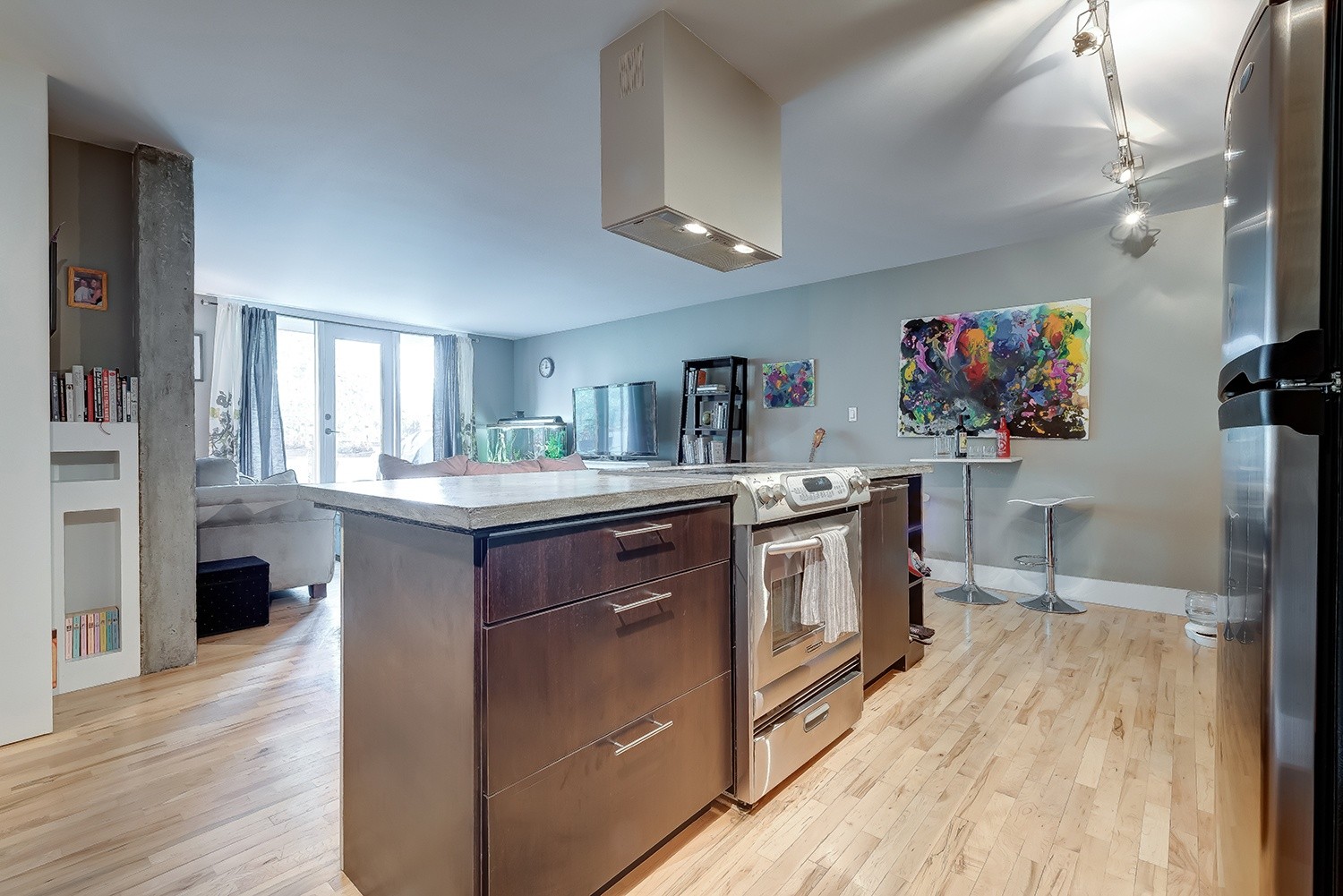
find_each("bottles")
[954,414,967,458]
[1184,590,1217,628]
[996,415,1010,458]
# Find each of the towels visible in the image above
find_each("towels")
[802,529,860,643]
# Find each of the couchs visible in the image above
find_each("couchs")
[195,457,336,598]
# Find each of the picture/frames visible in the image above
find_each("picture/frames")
[67,266,109,311]
[193,333,204,381]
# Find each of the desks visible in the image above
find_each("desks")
[910,456,1023,605]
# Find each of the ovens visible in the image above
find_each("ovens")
[724,504,863,811]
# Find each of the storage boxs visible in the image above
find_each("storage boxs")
[487,424,567,463]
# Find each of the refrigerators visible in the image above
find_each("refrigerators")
[1215,0,1343,896]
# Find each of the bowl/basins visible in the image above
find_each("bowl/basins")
[1184,622,1217,648]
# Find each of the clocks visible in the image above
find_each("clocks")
[539,358,554,378]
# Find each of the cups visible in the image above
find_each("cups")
[968,444,996,458]
[933,435,952,458]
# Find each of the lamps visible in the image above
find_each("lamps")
[1125,187,1151,227]
[1102,136,1143,185]
[1072,0,1111,59]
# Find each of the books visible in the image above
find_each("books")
[64,606,119,661]
[686,369,726,394]
[50,365,139,435]
[711,402,740,430]
[682,434,725,465]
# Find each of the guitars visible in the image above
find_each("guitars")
[809,428,827,462]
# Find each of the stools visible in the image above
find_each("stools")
[1004,496,1099,614]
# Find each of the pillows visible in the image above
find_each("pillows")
[239,469,298,485]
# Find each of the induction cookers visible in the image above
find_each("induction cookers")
[599,459,872,526]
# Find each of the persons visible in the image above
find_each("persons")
[75,278,102,305]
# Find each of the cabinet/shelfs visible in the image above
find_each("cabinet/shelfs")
[49,421,141,696]
[675,356,748,466]
[891,474,924,673]
[342,497,735,896]
